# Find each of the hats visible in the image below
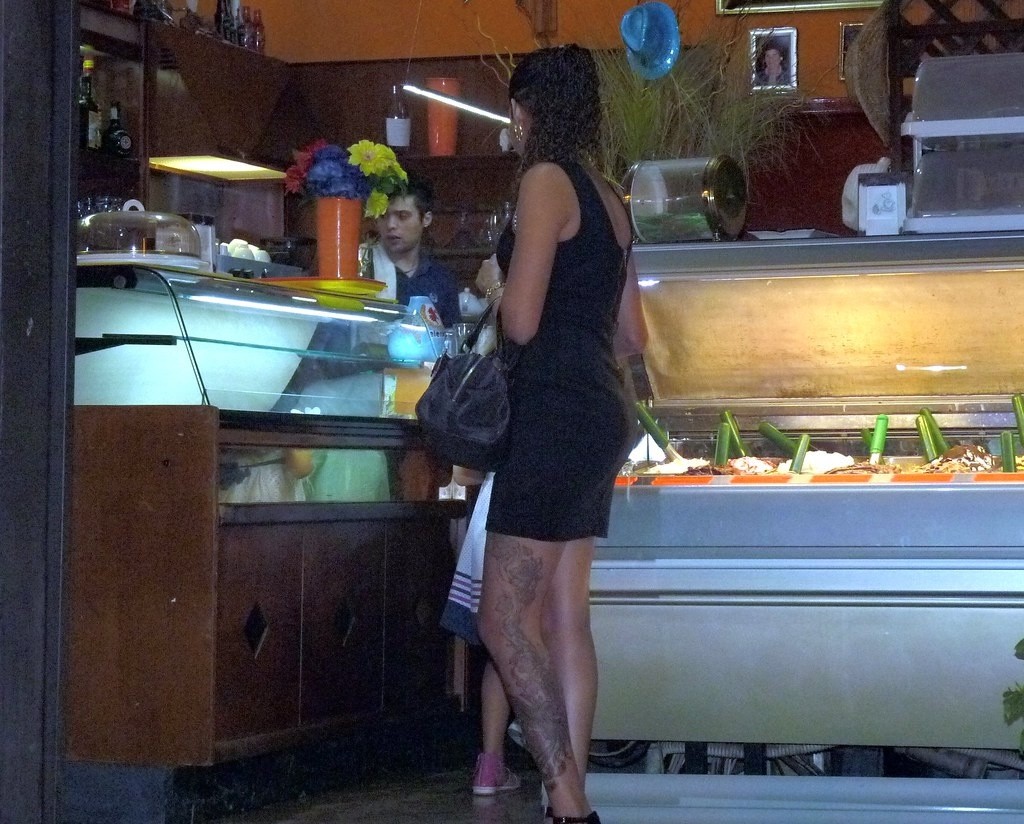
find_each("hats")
[759,41,785,63]
[845,1,889,147]
[620,1,681,80]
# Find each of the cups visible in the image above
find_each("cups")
[426,76,462,156]
[453,323,475,355]
[226,239,272,272]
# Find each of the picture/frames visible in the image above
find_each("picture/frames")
[747,27,798,96]
[716,0,885,14]
[839,21,865,80]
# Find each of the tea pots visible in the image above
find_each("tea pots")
[459,287,484,317]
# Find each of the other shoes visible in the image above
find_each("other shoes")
[543,807,600,824]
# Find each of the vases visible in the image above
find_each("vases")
[316,197,362,278]
[425,78,462,156]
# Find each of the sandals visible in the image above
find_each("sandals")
[472,753,520,793]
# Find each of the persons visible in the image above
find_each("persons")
[754,40,791,86]
[358,173,461,329]
[441,44,649,824]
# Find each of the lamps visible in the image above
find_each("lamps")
[397,0,511,124]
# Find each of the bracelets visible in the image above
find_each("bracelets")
[485,281,504,302]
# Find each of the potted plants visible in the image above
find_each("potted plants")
[386,101,411,146]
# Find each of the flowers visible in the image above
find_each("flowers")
[284,137,410,220]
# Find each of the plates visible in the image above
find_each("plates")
[747,228,840,239]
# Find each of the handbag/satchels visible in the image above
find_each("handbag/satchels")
[414,303,512,469]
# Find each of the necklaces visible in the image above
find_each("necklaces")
[400,265,419,276]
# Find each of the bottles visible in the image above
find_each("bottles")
[214,0,266,54]
[76,75,103,178]
[383,83,411,150]
[104,100,133,174]
